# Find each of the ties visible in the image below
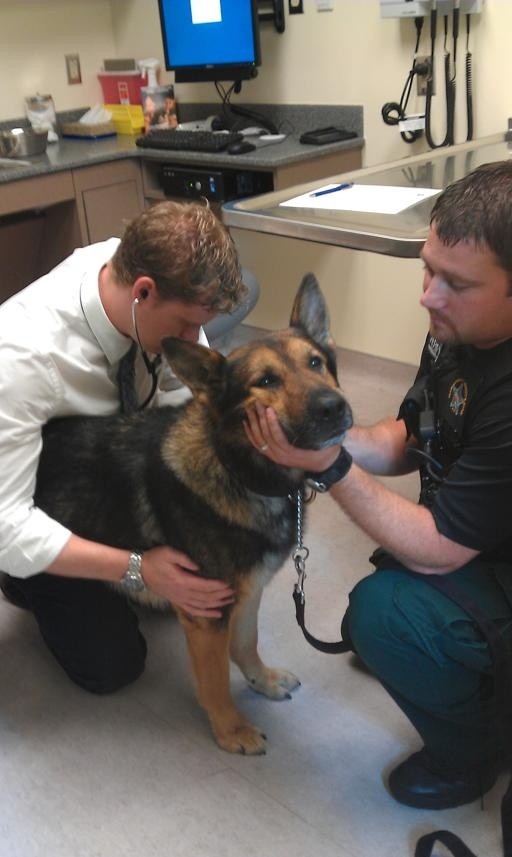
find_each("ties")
[119,340,138,413]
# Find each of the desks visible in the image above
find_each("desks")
[220,132,512,367]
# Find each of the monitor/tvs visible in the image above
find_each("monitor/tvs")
[157,0,261,84]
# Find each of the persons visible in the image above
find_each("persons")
[1,201,250,693]
[244,158,511,808]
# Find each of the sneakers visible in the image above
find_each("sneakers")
[389,742,511,810]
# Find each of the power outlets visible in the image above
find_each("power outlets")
[65,54,82,85]
[415,55,435,95]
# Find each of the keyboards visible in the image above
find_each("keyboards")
[136,128,243,153]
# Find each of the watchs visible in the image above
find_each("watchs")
[304,446,353,493]
[120,549,147,600]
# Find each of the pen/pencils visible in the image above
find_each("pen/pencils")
[310,182,353,197]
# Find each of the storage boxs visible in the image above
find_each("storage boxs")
[97,71,147,104]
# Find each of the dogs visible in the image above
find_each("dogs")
[33,271,354,755]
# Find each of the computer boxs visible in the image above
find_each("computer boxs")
[162,166,271,203]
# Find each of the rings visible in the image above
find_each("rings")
[260,444,269,452]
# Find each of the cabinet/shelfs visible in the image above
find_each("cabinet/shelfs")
[1,146,363,305]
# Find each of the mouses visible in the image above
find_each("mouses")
[226,141,257,154]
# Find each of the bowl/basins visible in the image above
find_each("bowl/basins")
[0,126,49,159]
